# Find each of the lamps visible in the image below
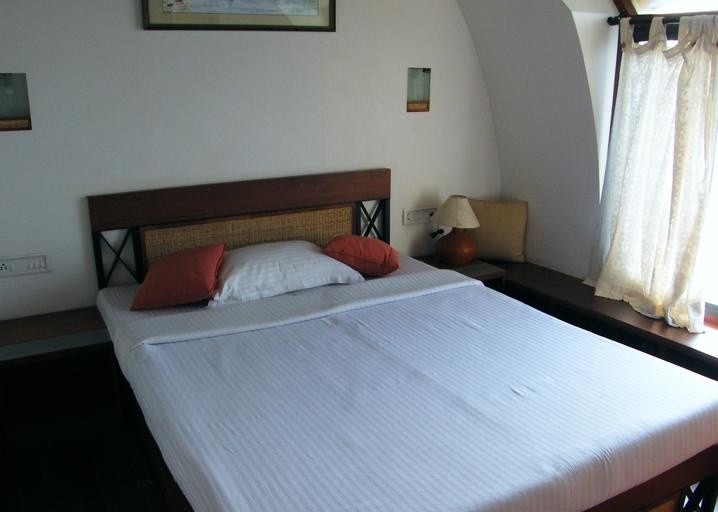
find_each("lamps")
[423,193,481,270]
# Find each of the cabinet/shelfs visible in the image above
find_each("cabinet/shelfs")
[498,255,717,378]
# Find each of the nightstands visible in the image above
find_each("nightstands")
[435,255,511,290]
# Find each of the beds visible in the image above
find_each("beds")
[82,162,717,510]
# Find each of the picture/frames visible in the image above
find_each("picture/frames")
[140,0,339,36]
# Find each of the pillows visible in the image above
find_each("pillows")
[459,193,530,267]
[127,239,227,311]
[203,237,369,310]
[324,230,400,281]
